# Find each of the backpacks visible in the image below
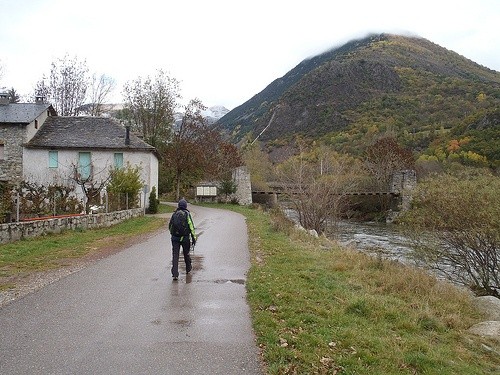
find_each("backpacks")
[170,211,191,237]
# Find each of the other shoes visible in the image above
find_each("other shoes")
[186,265,192,274]
[172,275,178,279]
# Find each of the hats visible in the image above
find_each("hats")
[178,199,188,209]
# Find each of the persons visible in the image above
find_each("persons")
[168,199,196,280]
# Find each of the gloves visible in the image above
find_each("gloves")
[191,239,196,246]
